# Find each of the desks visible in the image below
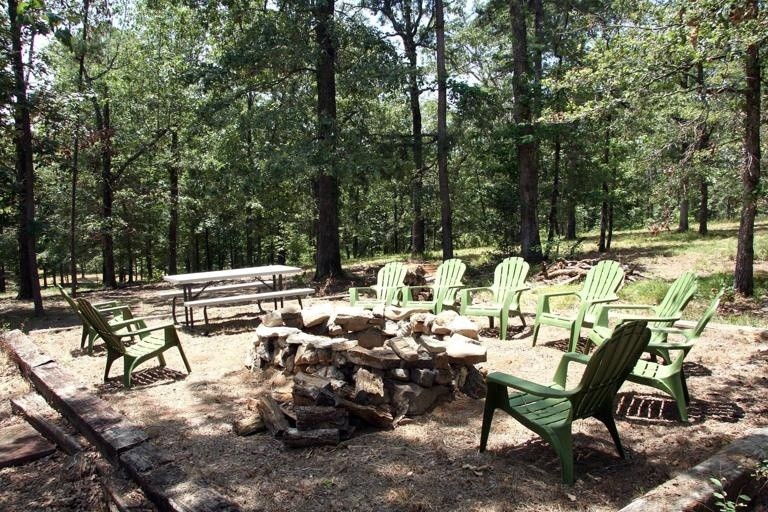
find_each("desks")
[163,264,301,327]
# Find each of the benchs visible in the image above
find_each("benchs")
[157,280,316,328]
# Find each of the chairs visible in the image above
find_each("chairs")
[476,318,653,486]
[579,269,699,366]
[347,261,408,305]
[552,286,725,421]
[392,257,467,316]
[461,256,531,341]
[530,259,627,354]
[54,282,192,388]
